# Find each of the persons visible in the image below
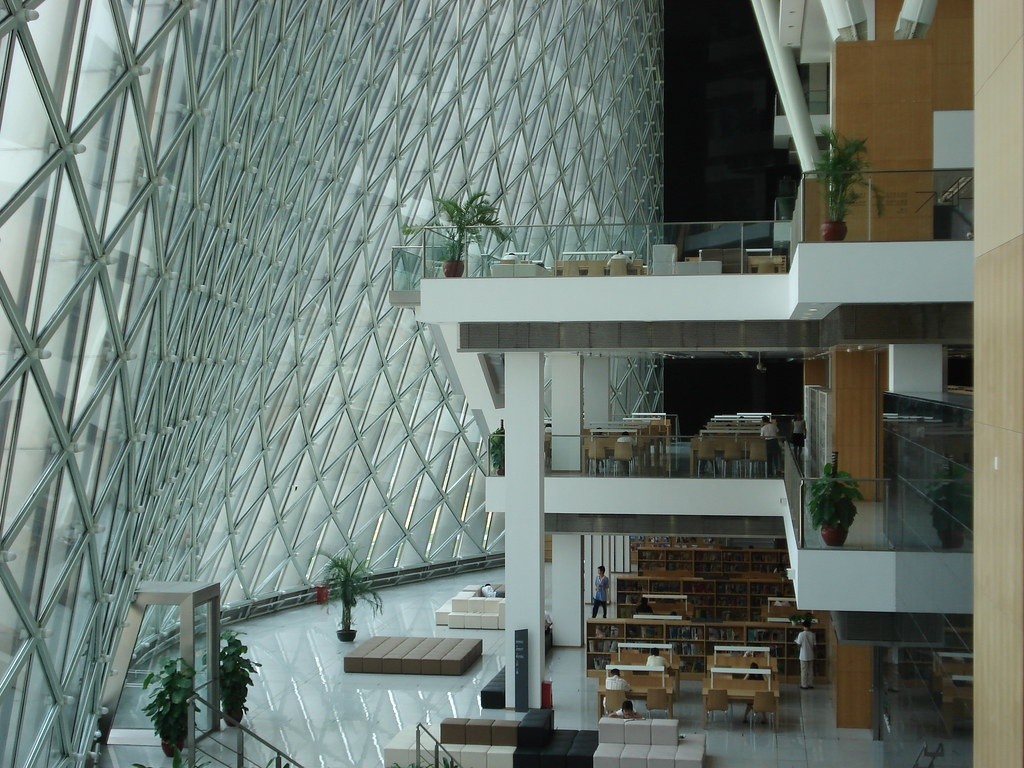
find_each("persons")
[790,412,807,461]
[697,426,720,474]
[636,597,654,614]
[743,662,769,723]
[646,648,672,673]
[794,620,816,690]
[616,431,637,476]
[606,250,633,267]
[760,416,779,476]
[603,668,630,716]
[592,566,611,619]
[545,424,552,459]
[482,583,505,598]
[743,651,769,658]
[593,427,604,475]
[608,701,644,719]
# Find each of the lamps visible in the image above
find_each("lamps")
[757,352,763,370]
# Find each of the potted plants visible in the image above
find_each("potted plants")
[813,124,883,242]
[489,428,505,475]
[201,630,263,726]
[306,545,384,642]
[141,658,202,758]
[401,190,512,277]
[806,463,865,547]
[924,456,973,547]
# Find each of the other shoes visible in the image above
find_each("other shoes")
[743,720,746,722]
[808,686,814,688]
[800,686,808,689]
[763,720,765,723]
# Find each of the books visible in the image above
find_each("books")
[590,535,826,677]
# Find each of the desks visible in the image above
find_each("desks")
[702,678,780,730]
[582,418,776,466]
[610,653,680,700]
[707,655,778,679]
[598,675,675,723]
[553,259,643,276]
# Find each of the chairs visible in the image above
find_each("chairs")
[610,259,627,277]
[660,651,674,655]
[500,260,514,264]
[698,440,716,478]
[758,666,775,681]
[587,262,604,276]
[614,442,633,478]
[748,440,768,477]
[650,670,669,677]
[646,688,673,719]
[587,445,607,475]
[705,689,729,727]
[714,665,732,680]
[614,664,632,676]
[622,649,639,654]
[564,261,580,277]
[544,432,551,462]
[749,692,775,730]
[721,440,742,478]
[606,690,626,715]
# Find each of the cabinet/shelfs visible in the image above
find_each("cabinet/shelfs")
[637,546,789,575]
[586,618,830,685]
[629,536,726,565]
[615,575,818,621]
